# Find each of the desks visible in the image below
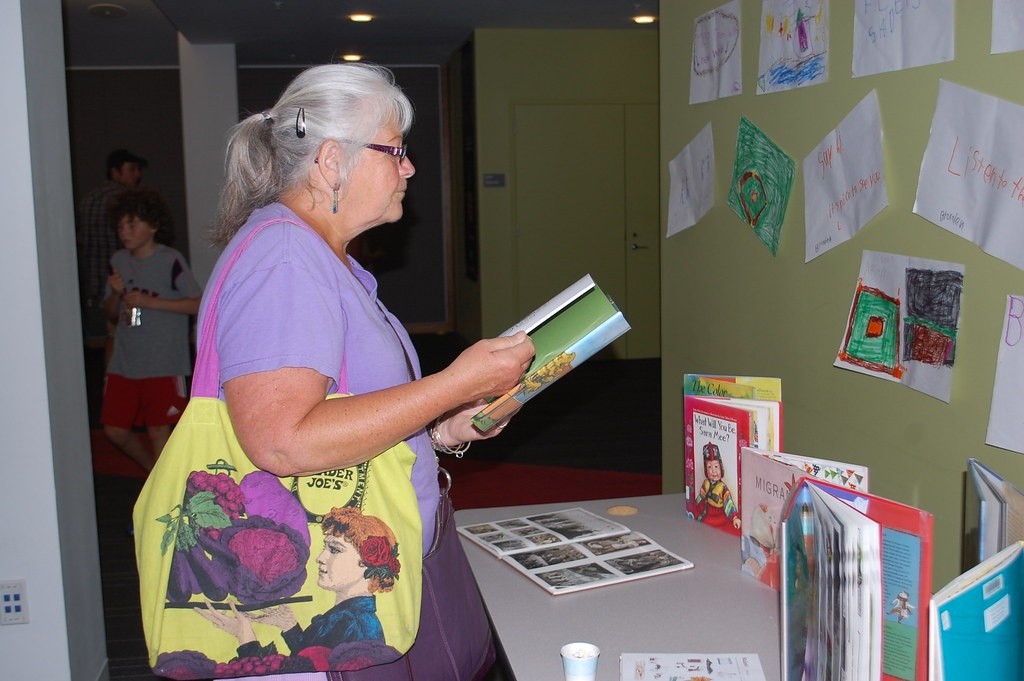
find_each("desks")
[454,492,783,681]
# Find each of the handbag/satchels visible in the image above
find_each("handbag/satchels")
[131,394,424,680]
[326,464,519,681]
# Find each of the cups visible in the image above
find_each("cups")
[123,305,142,327]
[560,642,600,681]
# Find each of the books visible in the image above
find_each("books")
[741,446,867,596]
[455,506,695,597]
[779,459,1024,681]
[681,375,781,537]
[472,272,629,433]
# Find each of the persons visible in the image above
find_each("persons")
[80,149,183,521]
[596,539,647,554]
[194,61,535,681]
[101,202,202,479]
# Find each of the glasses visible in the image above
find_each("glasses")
[341,137,408,162]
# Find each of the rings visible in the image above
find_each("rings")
[499,419,509,429]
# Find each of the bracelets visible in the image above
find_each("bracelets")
[430,416,471,458]
